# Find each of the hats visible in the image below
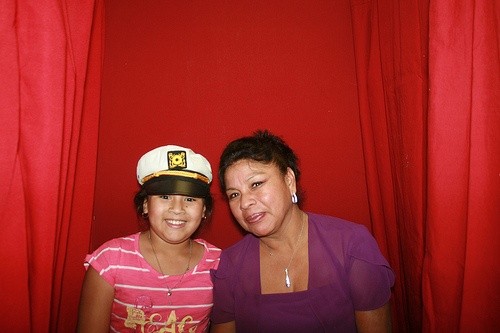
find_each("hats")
[137,145,213,197]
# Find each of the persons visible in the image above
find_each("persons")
[76,145,223,333]
[211,129,395,333]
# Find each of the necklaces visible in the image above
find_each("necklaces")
[263,212,306,287]
[149,231,192,296]
